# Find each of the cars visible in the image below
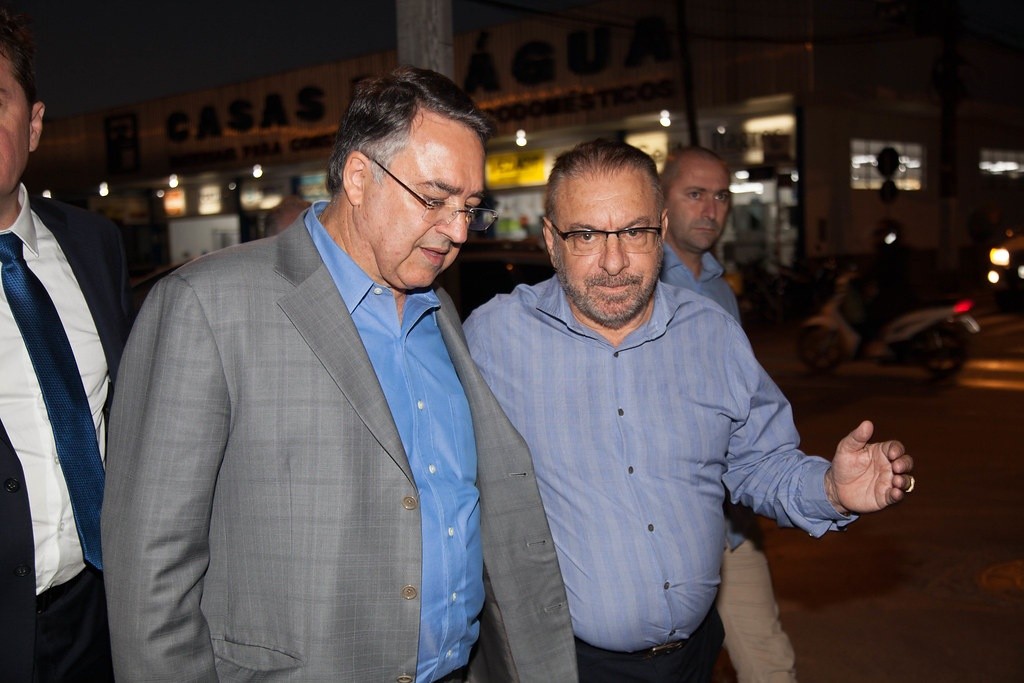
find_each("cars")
[990,224,1023,309]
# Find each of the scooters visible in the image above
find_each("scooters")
[791,245,981,385]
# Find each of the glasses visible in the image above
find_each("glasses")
[372,158,499,230]
[550,213,662,256]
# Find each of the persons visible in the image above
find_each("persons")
[0,2,143,683]
[99,63,580,683]
[854,218,915,360]
[463,135,915,682]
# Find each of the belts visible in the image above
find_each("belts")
[35,564,93,614]
[574,599,716,658]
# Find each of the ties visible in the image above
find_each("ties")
[0,232,106,570]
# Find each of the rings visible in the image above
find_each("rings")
[905,474,916,495]
